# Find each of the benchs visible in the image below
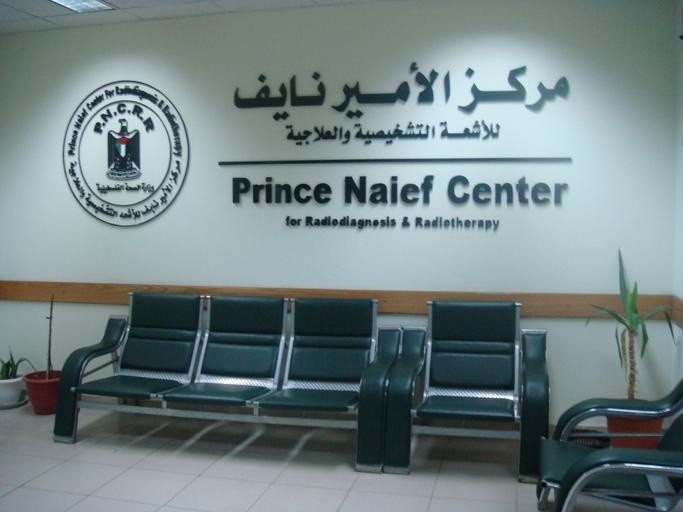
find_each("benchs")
[51,290,385,474]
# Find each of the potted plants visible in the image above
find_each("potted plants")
[589,251,683,451]
[1,295,65,416]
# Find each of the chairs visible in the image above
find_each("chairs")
[535,374,683,511]
[383,299,551,485]
